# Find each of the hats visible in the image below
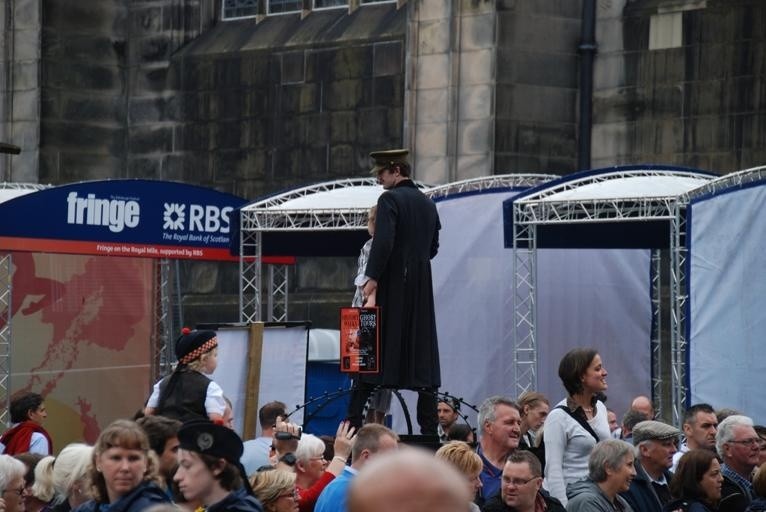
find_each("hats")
[175,327,217,363]
[369,149,411,174]
[632,420,681,444]
[178,421,245,464]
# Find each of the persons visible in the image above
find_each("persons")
[313,423,401,512]
[248,469,302,512]
[351,204,393,425]
[472,396,522,503]
[668,403,718,475]
[715,415,760,512]
[346,149,442,451]
[173,419,265,512]
[436,441,484,512]
[480,449,567,512]
[134,416,184,504]
[291,420,357,512]
[662,449,725,512]
[611,396,654,440]
[544,348,612,510]
[443,424,475,447]
[0,454,28,512]
[0,392,53,458]
[565,439,638,512]
[753,425,766,474]
[620,410,648,447]
[346,444,469,512]
[515,392,551,450]
[433,400,460,444]
[716,409,743,426]
[746,462,766,512]
[32,443,100,512]
[144,328,231,425]
[239,401,290,478]
[605,408,619,434]
[15,453,49,512]
[317,434,337,469]
[72,419,175,512]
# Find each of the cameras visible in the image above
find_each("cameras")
[272,424,301,440]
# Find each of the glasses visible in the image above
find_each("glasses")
[310,455,326,463]
[728,437,760,447]
[282,488,301,499]
[502,474,537,486]
